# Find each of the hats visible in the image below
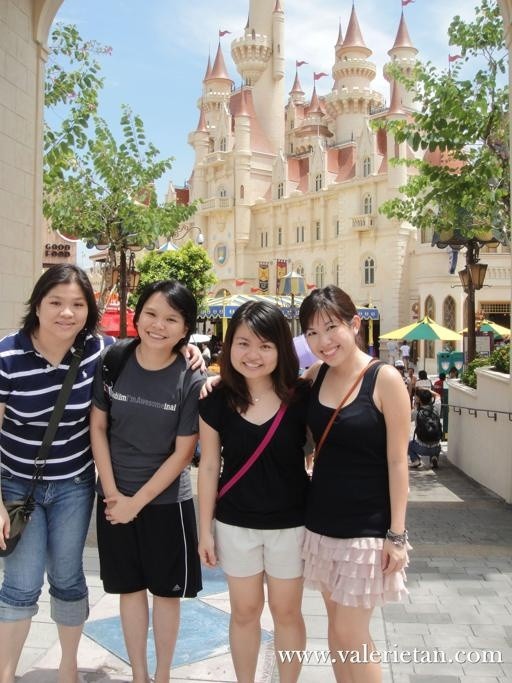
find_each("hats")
[394,360,405,368]
[416,389,432,402]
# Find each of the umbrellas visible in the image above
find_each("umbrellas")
[459,318,512,342]
[378,315,466,375]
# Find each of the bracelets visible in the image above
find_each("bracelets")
[384,529,411,548]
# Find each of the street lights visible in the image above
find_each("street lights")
[172,225,204,244]
[93,225,147,339]
[431,212,508,371]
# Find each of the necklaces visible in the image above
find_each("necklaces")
[242,384,277,404]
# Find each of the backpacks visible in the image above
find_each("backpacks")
[418,406,442,442]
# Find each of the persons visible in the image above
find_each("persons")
[196,296,316,680]
[0,261,208,682]
[387,340,464,470]
[197,288,412,682]
[88,276,206,681]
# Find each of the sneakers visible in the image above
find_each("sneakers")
[432,456,439,468]
[408,458,421,468]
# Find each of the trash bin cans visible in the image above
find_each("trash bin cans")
[437,352,464,376]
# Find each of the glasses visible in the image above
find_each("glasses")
[397,368,403,370]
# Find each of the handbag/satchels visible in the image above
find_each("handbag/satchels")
[0,499,35,557]
